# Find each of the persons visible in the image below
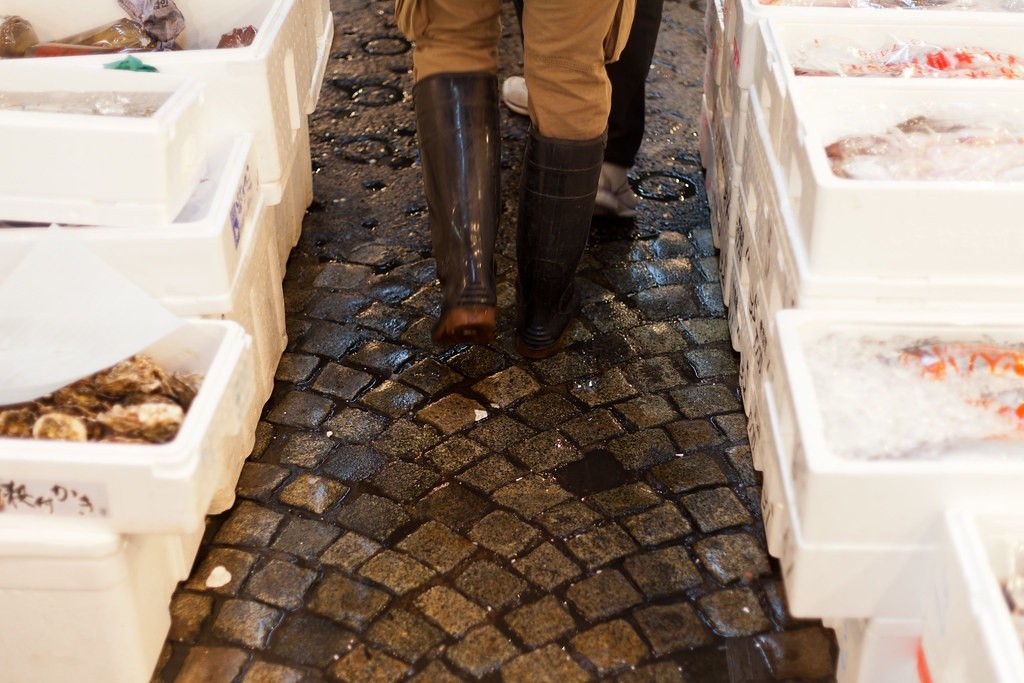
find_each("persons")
[503,1,663,216]
[394,1,638,359]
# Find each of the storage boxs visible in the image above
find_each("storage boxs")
[701,0,1024,683]
[0,0,335,683]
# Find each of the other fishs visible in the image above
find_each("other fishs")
[856,341,1023,462]
[789,47,1024,181]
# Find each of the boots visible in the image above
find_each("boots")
[514,124,610,358]
[412,69,500,345]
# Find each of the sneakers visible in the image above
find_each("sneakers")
[501,76,529,115]
[594,162,638,218]
